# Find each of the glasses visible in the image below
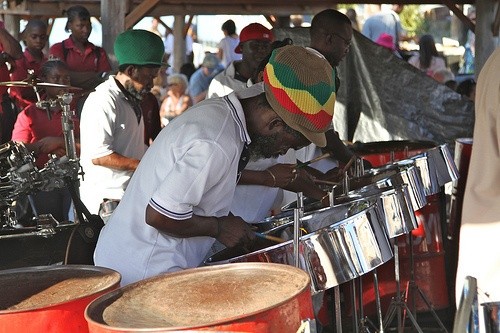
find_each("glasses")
[333,32,353,53]
[241,41,271,53]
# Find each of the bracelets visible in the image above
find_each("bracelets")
[266,167,280,185]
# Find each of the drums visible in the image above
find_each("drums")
[0,214,105,270]
[0,265,122,333]
[203,197,394,297]
[280,144,461,239]
[0,141,43,206]
[83,262,316,333]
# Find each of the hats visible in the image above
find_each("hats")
[202,54,218,70]
[262,43,337,148]
[112,29,171,68]
[169,73,190,87]
[360,10,404,60]
[234,22,274,54]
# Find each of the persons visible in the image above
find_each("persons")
[453,10,497,332]
[89,45,337,304]
[276,7,355,216]
[9,61,85,173]
[180,0,477,176]
[203,23,277,112]
[158,72,191,131]
[68,28,167,230]
[0,1,111,147]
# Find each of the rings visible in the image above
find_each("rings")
[288,179,295,183]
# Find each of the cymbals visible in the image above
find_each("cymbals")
[0,80,82,90]
